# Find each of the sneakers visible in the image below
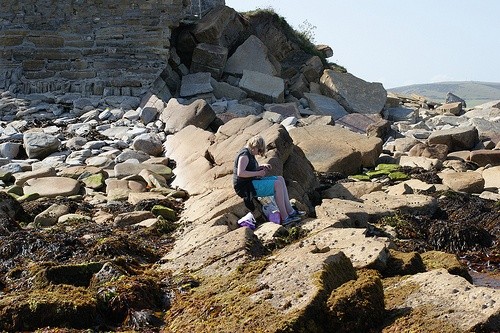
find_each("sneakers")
[258,149,261,152]
[281,216,301,227]
[289,210,306,217]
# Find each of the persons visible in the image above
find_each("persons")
[232,135,306,226]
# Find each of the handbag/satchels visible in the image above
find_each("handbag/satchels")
[263,199,281,225]
[238,211,256,229]
